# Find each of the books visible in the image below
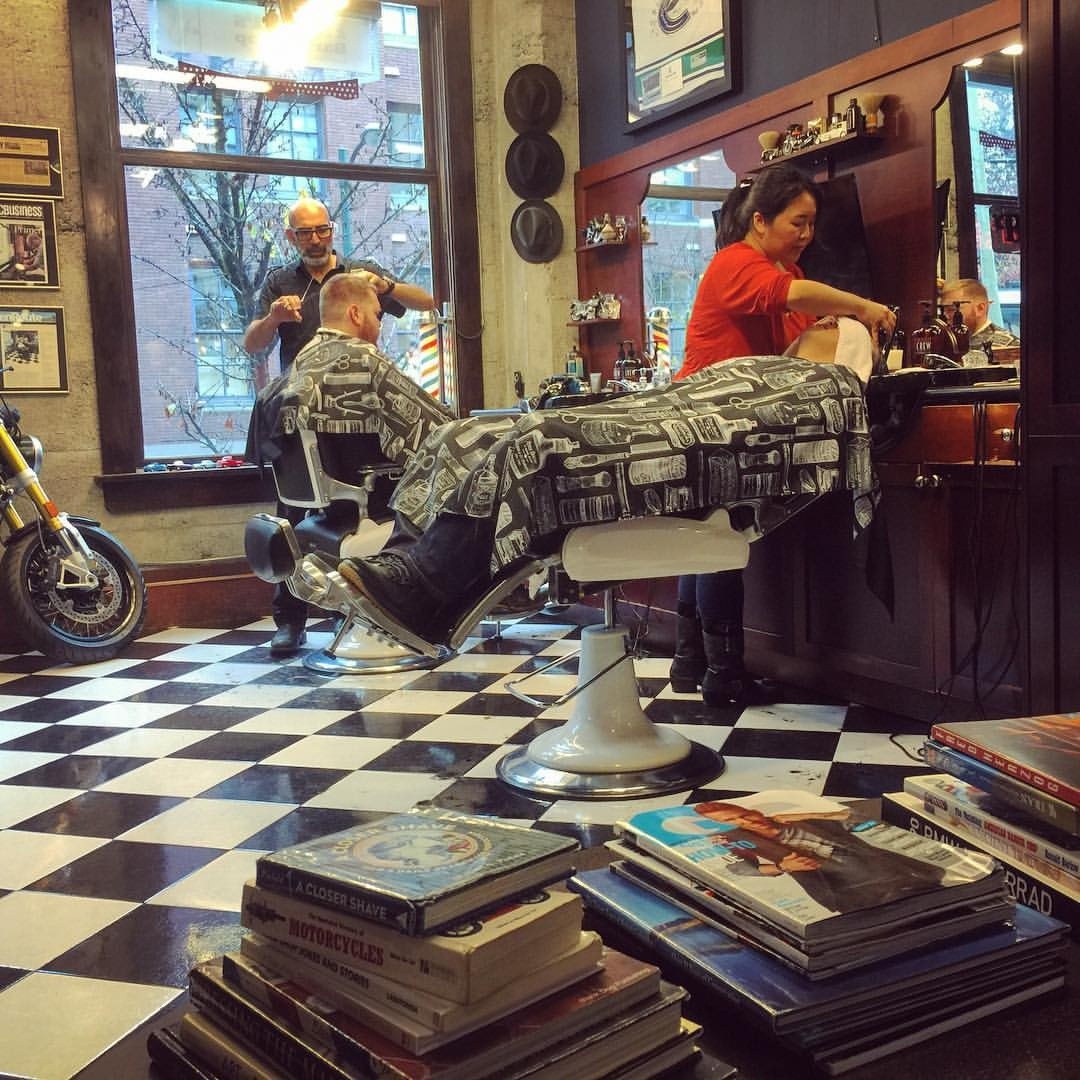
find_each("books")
[141,705,1080,1079]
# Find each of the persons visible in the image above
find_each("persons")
[339,306,882,654]
[937,277,1020,348]
[661,164,905,710]
[275,272,455,521]
[240,196,436,655]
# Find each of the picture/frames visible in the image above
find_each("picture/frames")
[0,121,71,395]
[619,1,741,132]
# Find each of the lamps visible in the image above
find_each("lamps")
[417,308,453,406]
[645,307,675,379]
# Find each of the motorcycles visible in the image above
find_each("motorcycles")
[0,363,150,662]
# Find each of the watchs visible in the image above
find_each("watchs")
[376,273,396,296]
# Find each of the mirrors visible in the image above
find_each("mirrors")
[927,36,1024,360]
[638,147,741,379]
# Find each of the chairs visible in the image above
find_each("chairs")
[244,384,926,801]
[245,404,462,673]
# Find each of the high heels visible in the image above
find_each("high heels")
[670,664,705,692]
[702,667,779,706]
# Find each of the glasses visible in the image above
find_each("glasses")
[290,222,333,241]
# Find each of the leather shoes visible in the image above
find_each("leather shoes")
[333,618,344,636]
[306,547,395,573]
[338,555,452,643]
[271,624,306,652]
